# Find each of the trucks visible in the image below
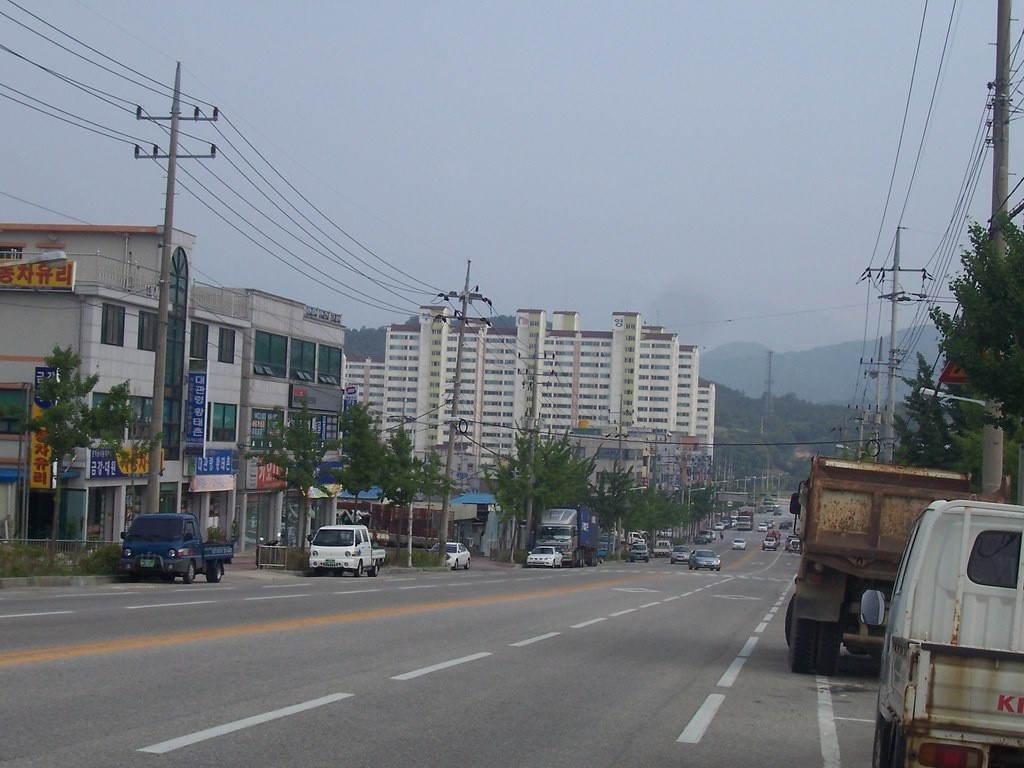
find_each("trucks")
[308,525,386,578]
[735,511,754,531]
[120,513,232,584]
[784,454,1009,675]
[860,499,1024,768]
[615,531,646,544]
[653,540,673,558]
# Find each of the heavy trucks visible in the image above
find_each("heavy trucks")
[537,506,599,568]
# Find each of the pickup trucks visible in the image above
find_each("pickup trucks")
[629,544,650,563]
[671,546,691,565]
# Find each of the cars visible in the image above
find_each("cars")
[689,516,747,573]
[757,500,801,552]
[526,546,563,568]
[427,541,471,570]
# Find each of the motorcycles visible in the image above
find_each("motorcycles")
[256,532,282,570]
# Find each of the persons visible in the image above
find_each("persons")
[720,531,723,536]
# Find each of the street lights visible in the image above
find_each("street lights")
[917,383,1024,507]
[687,487,705,516]
[612,486,647,554]
